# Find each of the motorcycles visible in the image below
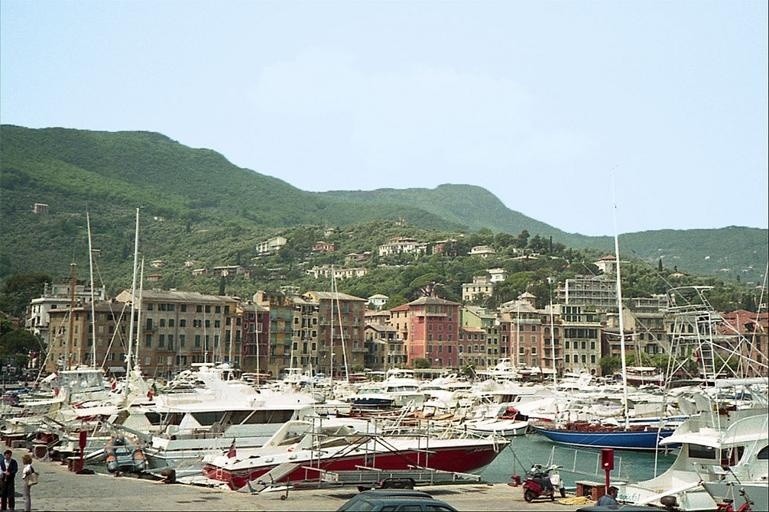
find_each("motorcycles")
[521,464,555,503]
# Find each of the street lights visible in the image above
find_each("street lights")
[22,472,39,487]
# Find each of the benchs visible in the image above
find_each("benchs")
[227,437,237,459]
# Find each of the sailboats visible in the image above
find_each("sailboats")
[529,167,684,454]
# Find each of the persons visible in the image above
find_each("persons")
[21,455,34,511]
[597,486,619,506]
[0,450,18,512]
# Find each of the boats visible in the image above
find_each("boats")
[603,311,769,512]
[199,415,511,491]
[0,204,769,480]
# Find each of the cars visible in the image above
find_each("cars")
[335,488,443,512]
[350,496,458,512]
